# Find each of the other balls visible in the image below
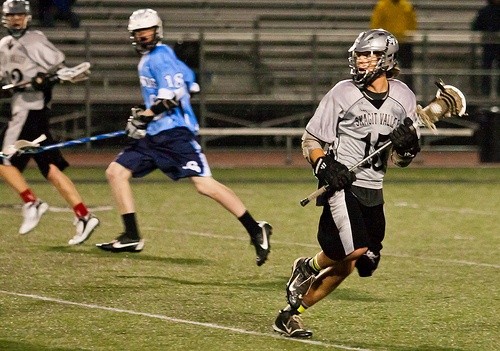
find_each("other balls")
[430,104,443,114]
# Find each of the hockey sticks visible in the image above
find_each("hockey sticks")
[300,84,467,208]
[2,61,91,89]
[0,128,128,159]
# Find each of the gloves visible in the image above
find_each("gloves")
[0,80,13,99]
[125,115,154,141]
[312,156,352,191]
[388,117,421,160]
[32,71,52,92]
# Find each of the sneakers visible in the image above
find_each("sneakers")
[95,232,145,253]
[18,199,49,235]
[284,257,316,309]
[68,213,100,245]
[272,309,312,339]
[250,221,273,266]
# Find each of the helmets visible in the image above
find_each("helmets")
[127,8,164,54]
[1,0,33,29]
[347,28,399,88]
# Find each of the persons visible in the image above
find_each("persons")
[370,0,418,93]
[0,0,99,246]
[94,9,272,267]
[470,0,500,97]
[272,28,421,340]
[37,0,80,30]
